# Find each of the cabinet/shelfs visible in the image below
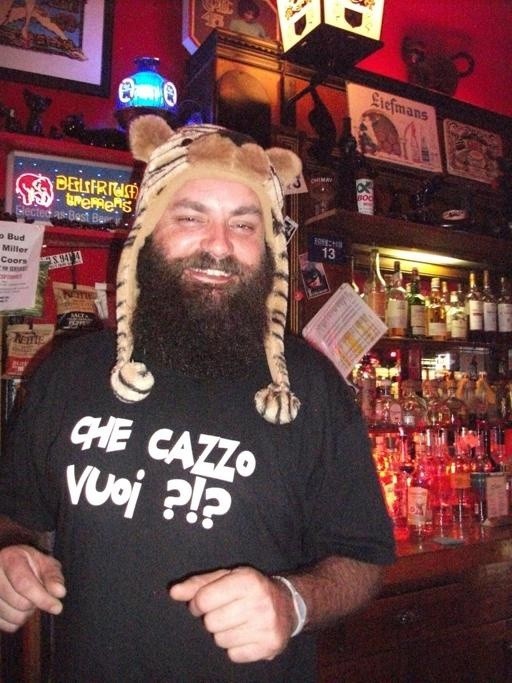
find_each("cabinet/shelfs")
[180,30,512,683]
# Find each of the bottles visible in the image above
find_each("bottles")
[386,260,410,337]
[497,274,512,330]
[483,272,496,333]
[358,353,512,544]
[427,278,449,342]
[467,271,488,333]
[449,290,465,342]
[363,247,387,334]
[407,266,428,339]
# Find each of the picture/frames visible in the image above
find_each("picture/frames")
[0,0,115,97]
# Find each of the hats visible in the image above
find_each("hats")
[105,115,305,426]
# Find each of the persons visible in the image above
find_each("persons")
[358,110,401,157]
[302,269,329,297]
[228,0,265,40]
[0,0,88,61]
[0,123,397,683]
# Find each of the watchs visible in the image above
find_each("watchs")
[270,576,307,638]
